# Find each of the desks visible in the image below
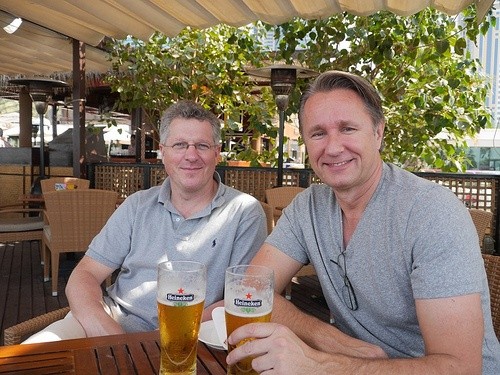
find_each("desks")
[17,192,126,205]
[0,330,228,375]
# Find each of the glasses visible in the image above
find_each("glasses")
[330,250,359,311]
[162,142,216,153]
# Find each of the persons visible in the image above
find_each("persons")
[0,128,6,148]
[224,69,499,375]
[19,99,268,347]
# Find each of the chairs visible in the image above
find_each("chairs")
[40,190,119,296]
[261,203,336,325]
[266,186,317,265]
[39,176,91,192]
[0,200,47,265]
[480,253,500,344]
[466,207,494,247]
[3,303,72,346]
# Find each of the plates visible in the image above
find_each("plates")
[196,318,228,351]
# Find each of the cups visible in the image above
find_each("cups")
[224,265,274,375]
[156,260,206,375]
